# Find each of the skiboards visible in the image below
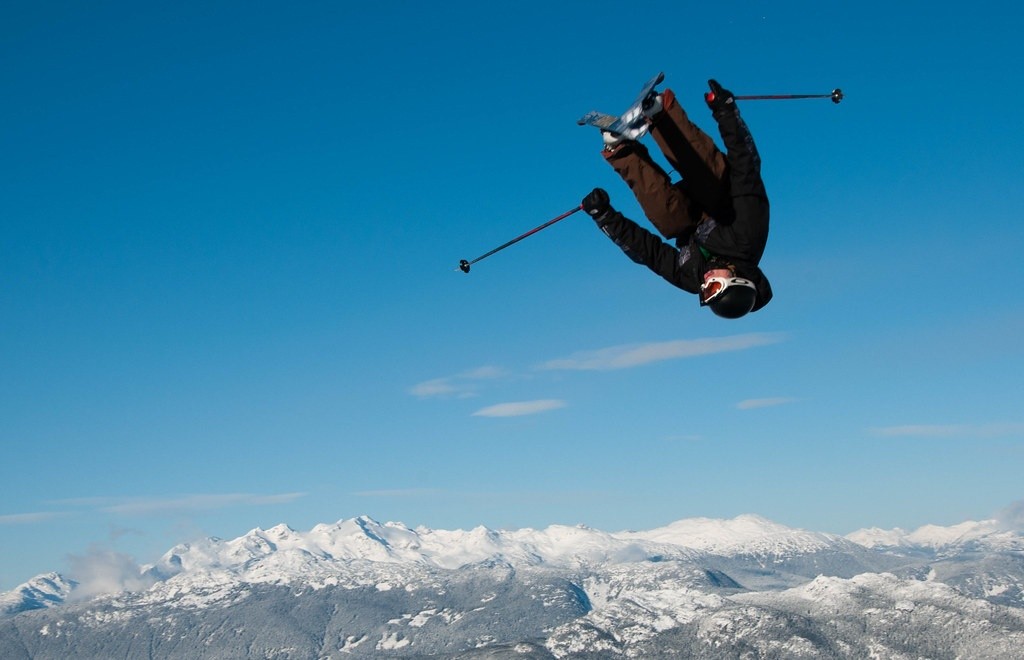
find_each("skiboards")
[576,68,669,143]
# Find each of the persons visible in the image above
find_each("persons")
[576,71,772,319]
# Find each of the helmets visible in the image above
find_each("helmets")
[709,277,756,319]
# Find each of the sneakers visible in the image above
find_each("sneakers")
[641,90,664,119]
[601,129,629,150]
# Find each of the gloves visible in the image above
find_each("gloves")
[581,187,615,229]
[704,79,737,116]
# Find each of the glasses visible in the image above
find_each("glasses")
[700,277,727,304]
